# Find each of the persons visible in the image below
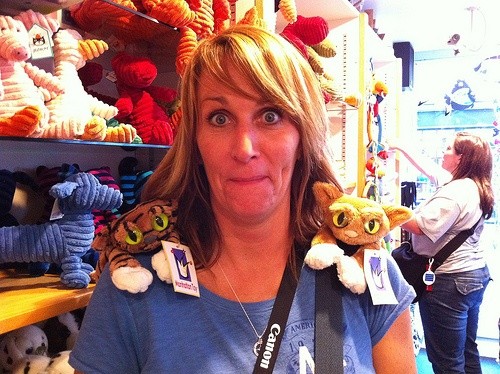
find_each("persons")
[399,132,495,374]
[68,27,417,374]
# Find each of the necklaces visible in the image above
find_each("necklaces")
[211,253,266,358]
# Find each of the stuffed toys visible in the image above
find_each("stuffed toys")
[0,0,413,374]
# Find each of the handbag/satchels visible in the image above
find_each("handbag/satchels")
[392,239,431,304]
